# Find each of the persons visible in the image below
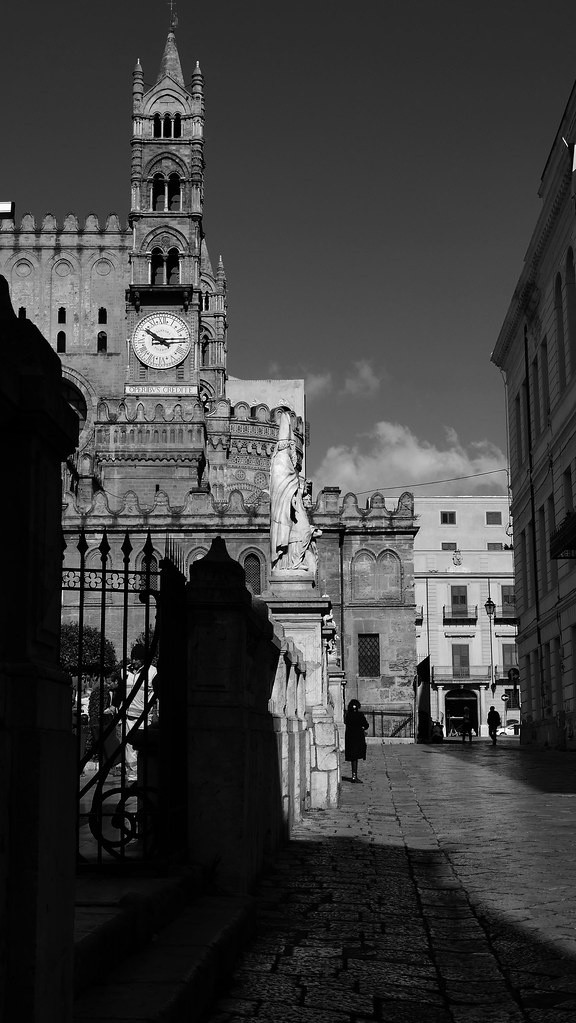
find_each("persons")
[462,707,473,745]
[487,706,501,747]
[432,722,443,743]
[345,699,369,784]
[270,412,322,572]
[76,643,157,782]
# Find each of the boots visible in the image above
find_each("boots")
[350,772,363,784]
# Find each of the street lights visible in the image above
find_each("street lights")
[484,597,496,688]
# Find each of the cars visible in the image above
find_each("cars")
[496,723,520,735]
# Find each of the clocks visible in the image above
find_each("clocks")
[132,309,193,370]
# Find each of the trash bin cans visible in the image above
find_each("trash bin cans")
[430,721,443,742]
